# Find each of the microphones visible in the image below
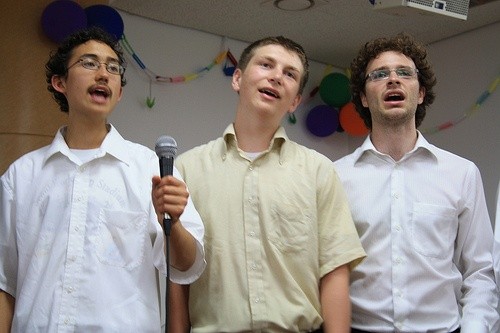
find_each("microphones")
[155,135,178,235]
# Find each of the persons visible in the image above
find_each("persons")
[0,27,207,333]
[333,35,500,333]
[166,35,368,333]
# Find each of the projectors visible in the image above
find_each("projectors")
[372,0,469,21]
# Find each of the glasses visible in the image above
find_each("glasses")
[363,66,419,85]
[66,58,126,75]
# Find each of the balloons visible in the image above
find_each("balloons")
[339,102,369,137]
[306,105,338,137]
[320,72,352,109]
[41,0,124,44]
